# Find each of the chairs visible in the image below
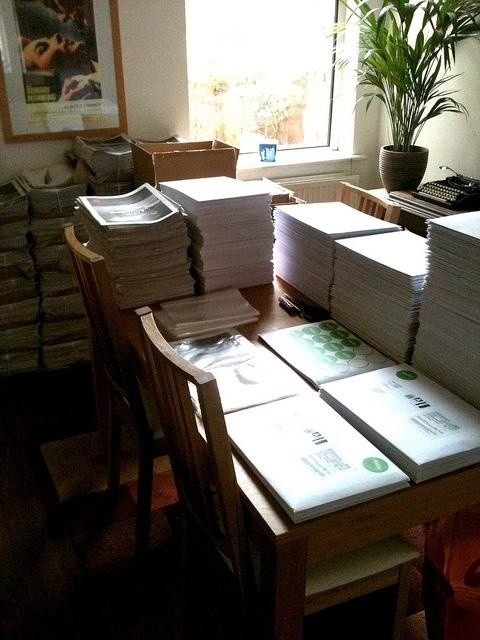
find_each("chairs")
[61,219,169,549]
[130,304,417,640]
[335,181,401,224]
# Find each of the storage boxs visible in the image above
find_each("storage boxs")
[130,138,240,191]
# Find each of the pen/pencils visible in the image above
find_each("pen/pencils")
[278,296,301,315]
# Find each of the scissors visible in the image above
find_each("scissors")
[284,292,330,323]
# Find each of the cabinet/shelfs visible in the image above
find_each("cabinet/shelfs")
[366,189,427,239]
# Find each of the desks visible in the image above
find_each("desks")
[118,279,480,640]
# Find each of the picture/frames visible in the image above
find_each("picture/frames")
[0,1,128,143]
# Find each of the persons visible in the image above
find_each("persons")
[15,32,103,102]
[41,0,96,101]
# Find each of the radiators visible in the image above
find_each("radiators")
[272,172,359,203]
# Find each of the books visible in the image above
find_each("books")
[72,174,274,309]
[2,132,137,372]
[243,179,479,351]
[151,286,260,338]
[167,319,480,525]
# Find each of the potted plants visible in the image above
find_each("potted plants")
[323,0,480,195]
